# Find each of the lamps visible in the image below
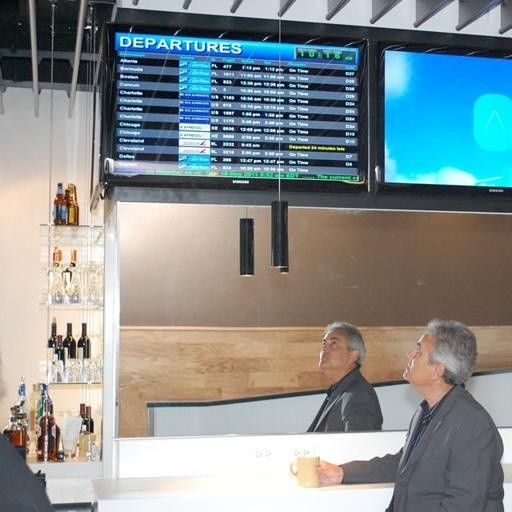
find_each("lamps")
[237,0,292,280]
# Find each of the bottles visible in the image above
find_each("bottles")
[3,181,93,463]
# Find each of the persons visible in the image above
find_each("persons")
[314,316,507,512]
[303,317,385,435]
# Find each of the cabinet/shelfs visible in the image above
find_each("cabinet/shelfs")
[33,221,104,489]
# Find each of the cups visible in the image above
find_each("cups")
[289,455,321,489]
[84,358,101,385]
